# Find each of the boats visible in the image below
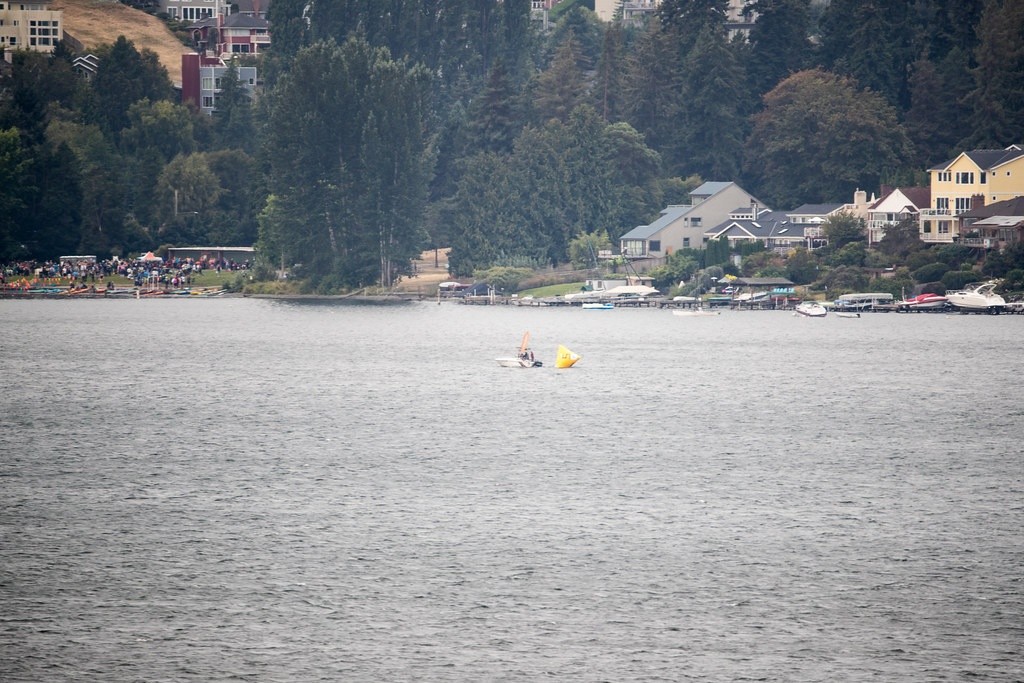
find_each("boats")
[581,302,615,310]
[947,284,1013,316]
[794,301,827,318]
[495,347,541,371]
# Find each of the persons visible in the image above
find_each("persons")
[523,348,529,360]
[0,256,253,294]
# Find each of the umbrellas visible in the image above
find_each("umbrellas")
[141,251,162,262]
